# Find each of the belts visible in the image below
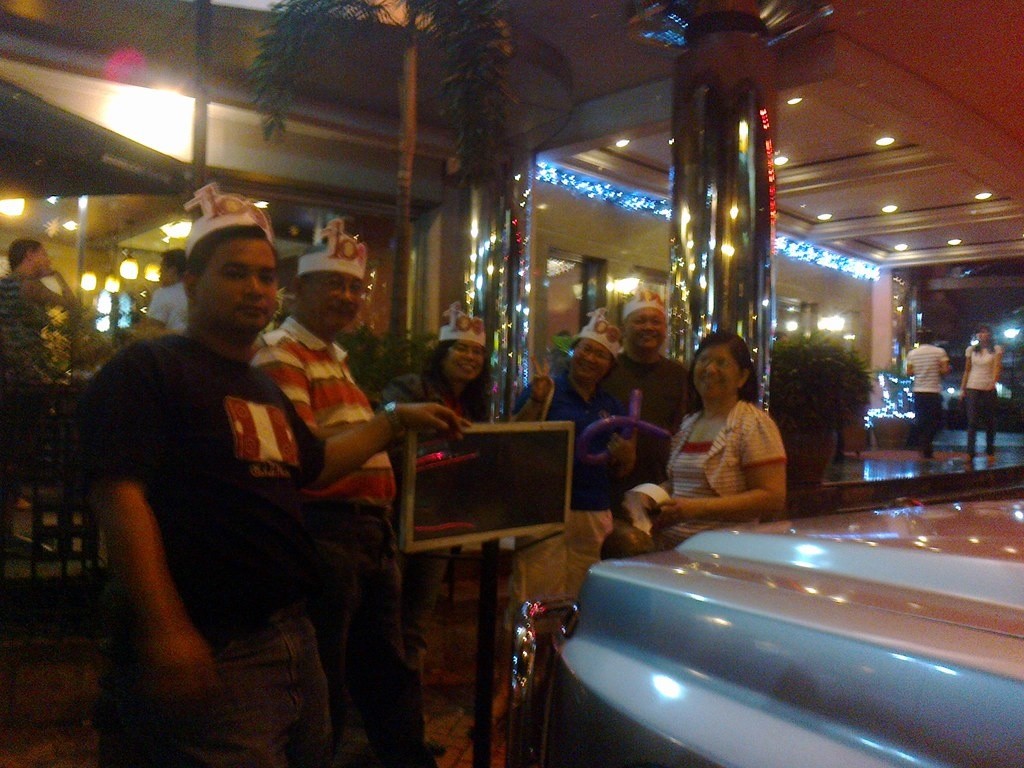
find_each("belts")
[300,498,387,521]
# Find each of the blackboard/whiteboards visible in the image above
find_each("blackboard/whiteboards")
[397,420,576,553]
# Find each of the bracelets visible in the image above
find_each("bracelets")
[384,400,403,432]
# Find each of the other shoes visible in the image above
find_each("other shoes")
[1,533,55,559]
[6,495,34,511]
[428,740,446,756]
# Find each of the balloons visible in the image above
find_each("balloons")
[576,389,672,465]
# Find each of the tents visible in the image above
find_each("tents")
[1,76,192,378]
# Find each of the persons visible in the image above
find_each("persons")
[0,238,80,548]
[81,184,786,768]
[961,323,1003,463]
[906,328,952,456]
[137,249,190,333]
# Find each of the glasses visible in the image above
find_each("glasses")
[449,342,489,358]
[696,354,734,369]
[575,342,609,360]
